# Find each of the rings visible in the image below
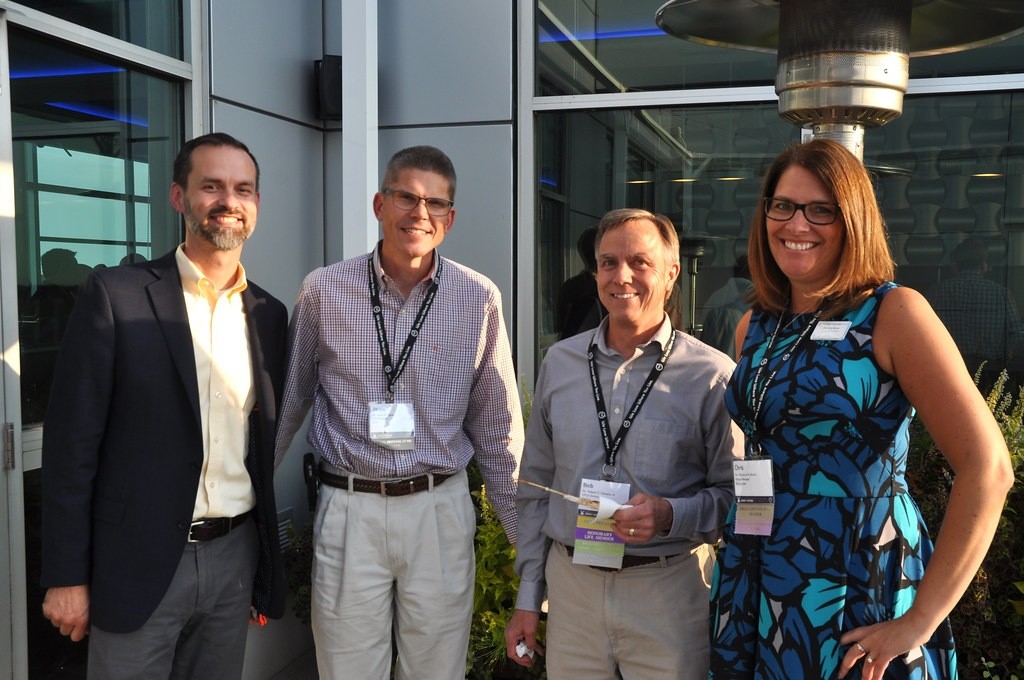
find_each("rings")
[629,528,635,536]
[867,654,876,664]
[857,642,866,653]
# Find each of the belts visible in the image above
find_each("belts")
[564,544,683,571]
[186,510,251,543]
[318,472,456,497]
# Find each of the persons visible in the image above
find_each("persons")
[31,248,149,410]
[710,140,1015,680]
[275,144,524,680]
[506,208,736,680]
[40,132,289,680]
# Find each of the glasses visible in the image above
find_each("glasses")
[761,196,841,226]
[384,188,454,217]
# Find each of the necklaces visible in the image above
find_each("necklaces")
[781,301,816,331]
